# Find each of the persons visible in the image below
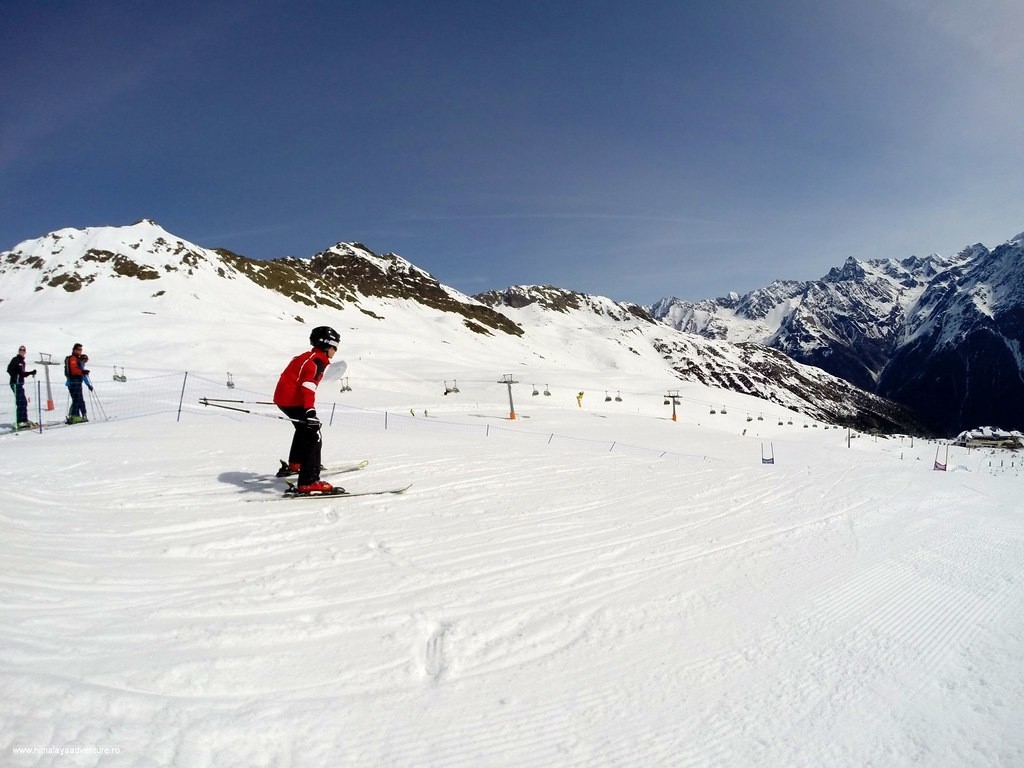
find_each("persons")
[7,346,36,427]
[65,354,93,418]
[65,344,90,422]
[274,326,341,491]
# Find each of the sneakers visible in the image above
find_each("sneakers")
[288,461,327,472]
[20,421,32,427]
[70,415,82,421]
[295,481,332,495]
[83,414,87,421]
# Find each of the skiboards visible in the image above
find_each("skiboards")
[278,459,413,503]
[0,422,62,436]
[44,415,117,430]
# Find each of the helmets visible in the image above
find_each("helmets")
[310,327,341,351]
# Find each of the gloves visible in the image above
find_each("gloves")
[305,407,320,431]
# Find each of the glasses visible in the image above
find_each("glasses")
[19,348,25,351]
[76,348,82,351]
